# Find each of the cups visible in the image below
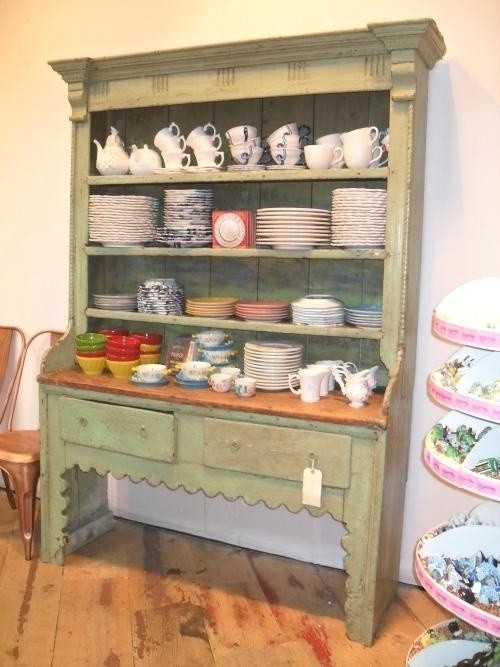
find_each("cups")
[154,122,389,169]
[211,367,256,397]
[288,358,344,402]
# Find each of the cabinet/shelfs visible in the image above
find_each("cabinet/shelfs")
[38,17,446,648]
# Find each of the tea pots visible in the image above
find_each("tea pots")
[93,125,163,175]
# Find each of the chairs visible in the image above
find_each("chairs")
[0,330,65,562]
[0,326,26,433]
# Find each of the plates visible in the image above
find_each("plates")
[156,188,214,248]
[330,187,386,249]
[242,338,305,393]
[175,373,209,388]
[153,164,306,174]
[88,195,161,247]
[129,374,169,387]
[214,214,246,247]
[91,278,382,331]
[256,207,331,249]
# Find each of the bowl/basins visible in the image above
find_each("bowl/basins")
[74,329,163,379]
[191,330,239,363]
[131,363,171,382]
[210,362,238,371]
[175,361,215,381]
